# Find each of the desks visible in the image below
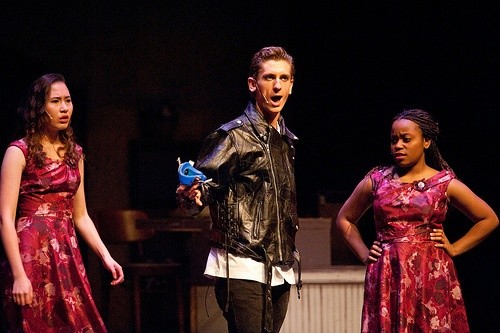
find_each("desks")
[186,265,367,333]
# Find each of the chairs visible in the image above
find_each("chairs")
[99,209,187,333]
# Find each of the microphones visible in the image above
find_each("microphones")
[45,111,53,120]
[256,84,268,104]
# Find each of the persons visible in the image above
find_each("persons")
[0,73,124,333]
[176,47,301,333]
[336,109,500,333]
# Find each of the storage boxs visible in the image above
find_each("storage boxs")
[291,218,334,267]
